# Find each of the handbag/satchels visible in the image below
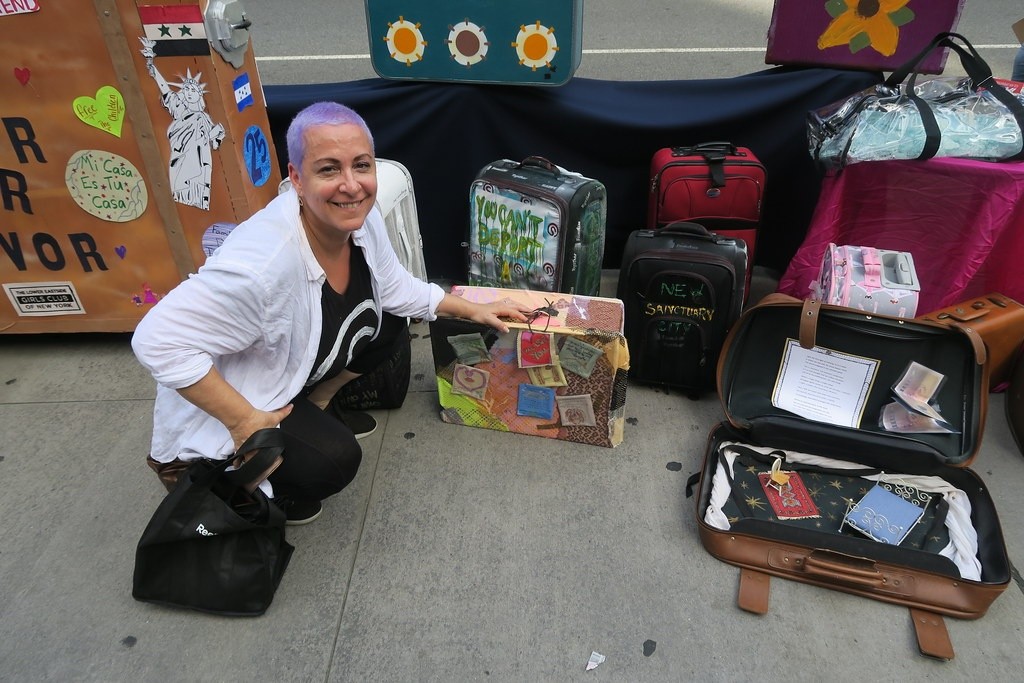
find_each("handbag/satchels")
[807,31,1024,171]
[132,428,295,617]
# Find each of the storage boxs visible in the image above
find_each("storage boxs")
[764,0,966,75]
[364,0,584,88]
[913,292,1024,391]
[0,0,283,337]
[428,284,631,449]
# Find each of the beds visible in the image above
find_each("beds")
[837,470,933,547]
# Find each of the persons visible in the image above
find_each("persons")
[131,102,535,526]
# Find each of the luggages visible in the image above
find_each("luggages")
[467,156,606,295]
[808,243,920,318]
[651,142,767,299]
[616,221,747,394]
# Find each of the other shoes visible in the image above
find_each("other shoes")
[273,492,323,525]
[327,407,378,441]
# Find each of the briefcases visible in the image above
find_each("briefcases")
[429,286,633,448]
[907,291,1024,389]
[687,287,1011,663]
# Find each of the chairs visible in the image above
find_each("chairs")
[765,457,791,496]
